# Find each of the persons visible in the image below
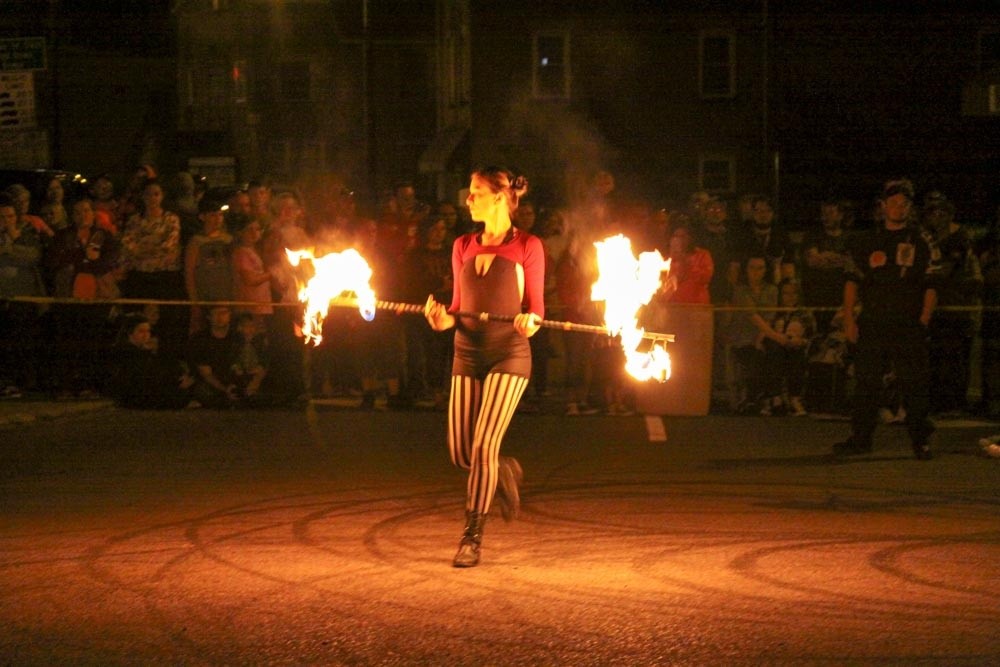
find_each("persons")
[922,190,1000,416]
[834,181,944,458]
[425,169,545,568]
[514,171,907,423]
[0,161,485,408]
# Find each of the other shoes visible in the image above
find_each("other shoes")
[784,397,808,416]
[978,434,1000,461]
[386,395,416,411]
[760,397,784,416]
[877,405,909,425]
[910,439,929,461]
[0,385,23,400]
[361,391,378,410]
[832,436,874,456]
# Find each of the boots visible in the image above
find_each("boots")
[454,510,488,567]
[493,453,523,521]
